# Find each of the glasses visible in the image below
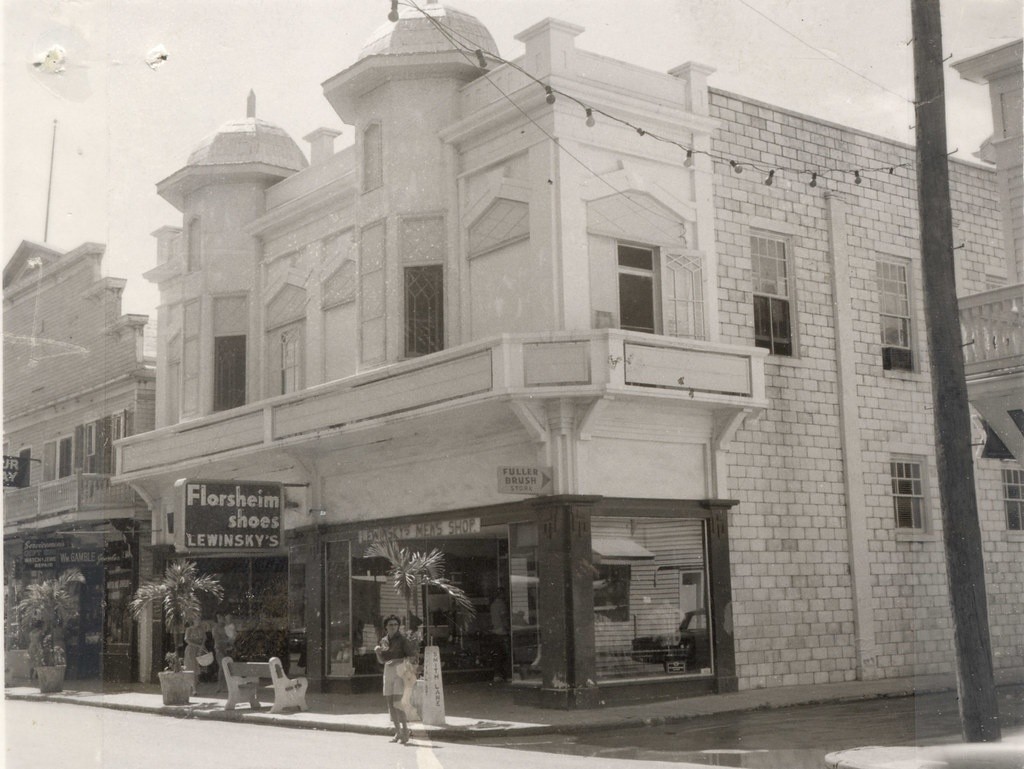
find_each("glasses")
[388,622,400,628]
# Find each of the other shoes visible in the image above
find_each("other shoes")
[390,732,400,743]
[193,691,197,695]
[400,730,413,743]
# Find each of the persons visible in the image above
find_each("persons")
[492,587,512,681]
[212,612,237,692]
[29,620,54,683]
[374,615,415,744]
[185,615,206,695]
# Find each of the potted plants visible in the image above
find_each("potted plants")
[130,557,225,705]
[12,567,87,693]
[363,525,477,722]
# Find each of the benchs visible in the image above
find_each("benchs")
[221,656,309,713]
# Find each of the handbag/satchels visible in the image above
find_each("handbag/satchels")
[196,648,214,667]
